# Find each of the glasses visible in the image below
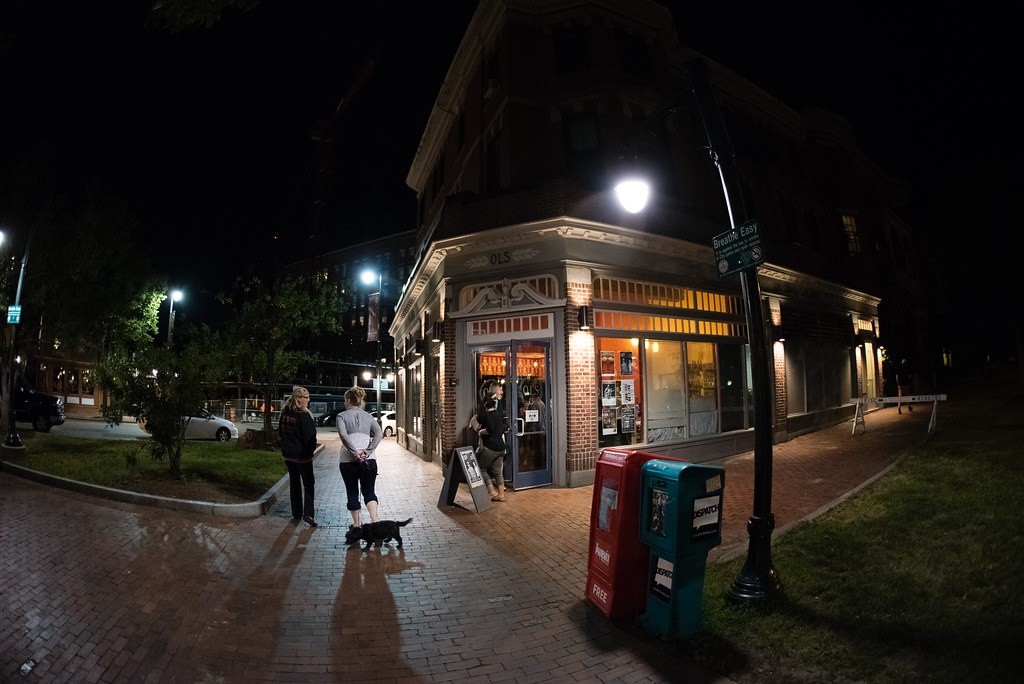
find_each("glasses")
[302,396,310,400]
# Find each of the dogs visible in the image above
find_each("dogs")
[344,517,413,552]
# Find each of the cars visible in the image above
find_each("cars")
[370,410,397,437]
[138,407,239,442]
[316,409,345,427]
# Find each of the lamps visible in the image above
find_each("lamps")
[777,326,786,342]
[431,322,441,342]
[580,306,589,329]
[415,340,422,356]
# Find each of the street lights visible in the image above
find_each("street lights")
[611,87,787,609]
[358,268,382,428]
[0,219,26,453]
[167,289,184,341]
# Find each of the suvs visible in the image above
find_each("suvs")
[12,372,66,432]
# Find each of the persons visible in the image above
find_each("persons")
[604,384,614,398]
[517,385,545,466]
[476,382,509,502]
[279,387,318,527]
[602,354,614,371]
[339,387,383,527]
[621,357,632,375]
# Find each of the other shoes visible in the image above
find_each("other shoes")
[487,491,505,502]
[293,514,318,527]
